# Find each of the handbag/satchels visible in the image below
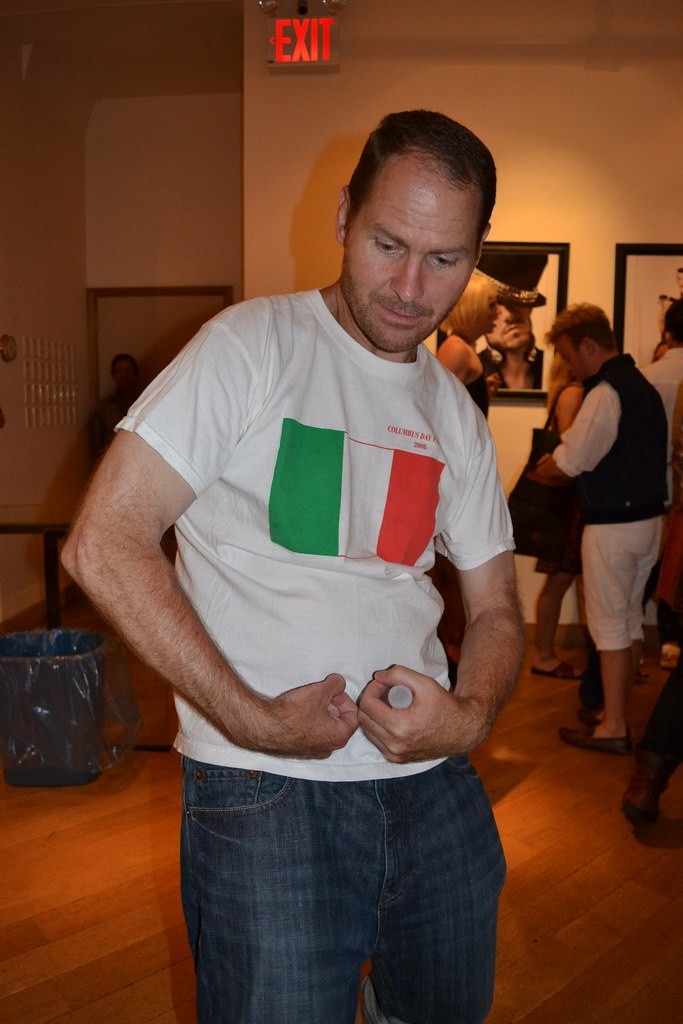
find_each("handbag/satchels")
[505,382,581,565]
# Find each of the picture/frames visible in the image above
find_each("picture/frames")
[436,241,570,402]
[613,242,683,356]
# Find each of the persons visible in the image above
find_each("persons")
[474,302,544,389]
[532,351,652,680]
[639,299,683,669]
[93,353,144,456]
[622,375,683,819]
[61,107,527,1024]
[536,305,669,755]
[651,341,670,362]
[430,275,501,683]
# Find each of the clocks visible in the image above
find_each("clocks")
[0,335,18,362]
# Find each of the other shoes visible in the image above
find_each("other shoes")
[619,765,669,823]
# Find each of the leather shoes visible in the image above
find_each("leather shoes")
[557,706,633,756]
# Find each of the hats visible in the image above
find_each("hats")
[474,252,549,307]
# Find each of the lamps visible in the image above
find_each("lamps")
[258,0,348,18]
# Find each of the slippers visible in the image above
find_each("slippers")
[531,659,583,678]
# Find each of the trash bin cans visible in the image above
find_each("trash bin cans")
[0,628,145,787]
